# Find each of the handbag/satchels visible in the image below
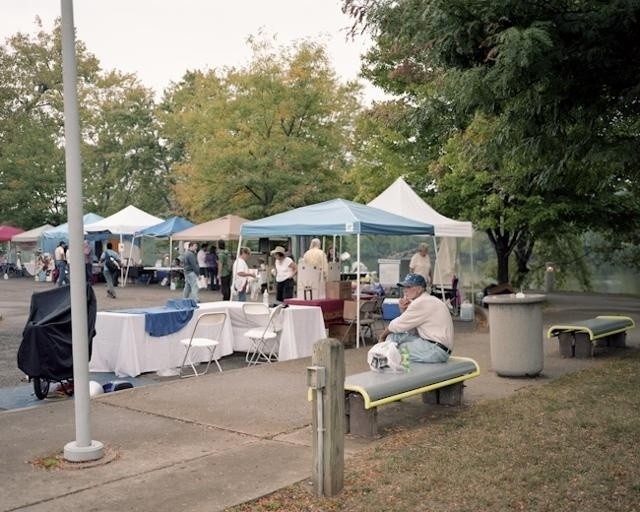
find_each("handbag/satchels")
[105,251,121,272]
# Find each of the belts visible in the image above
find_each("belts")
[428,339,452,355]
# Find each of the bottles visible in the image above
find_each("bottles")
[263,290,269,308]
[400,345,409,372]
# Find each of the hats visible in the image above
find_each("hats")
[397,272,427,289]
[270,246,286,255]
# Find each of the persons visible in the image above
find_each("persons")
[303,238,328,273]
[216,240,232,301]
[182,242,200,303]
[172,258,183,268]
[270,246,298,308]
[232,247,256,301]
[409,243,433,294]
[378,273,454,364]
[205,246,220,290]
[83,240,95,285]
[197,244,208,275]
[326,245,340,264]
[100,243,121,298]
[55,241,70,286]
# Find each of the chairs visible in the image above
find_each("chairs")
[248,303,283,369]
[341,300,378,348]
[242,302,285,363]
[178,311,228,380]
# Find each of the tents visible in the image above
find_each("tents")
[10,224,53,280]
[83,204,166,287]
[0,226,23,245]
[39,213,104,283]
[124,215,195,289]
[236,199,448,349]
[172,215,253,242]
[367,177,477,328]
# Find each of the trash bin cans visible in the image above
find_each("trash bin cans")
[483,293,547,377]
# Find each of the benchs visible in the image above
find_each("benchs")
[343,356,481,438]
[546,314,636,360]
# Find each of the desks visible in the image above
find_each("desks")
[89,304,234,378]
[283,297,356,327]
[220,301,330,362]
